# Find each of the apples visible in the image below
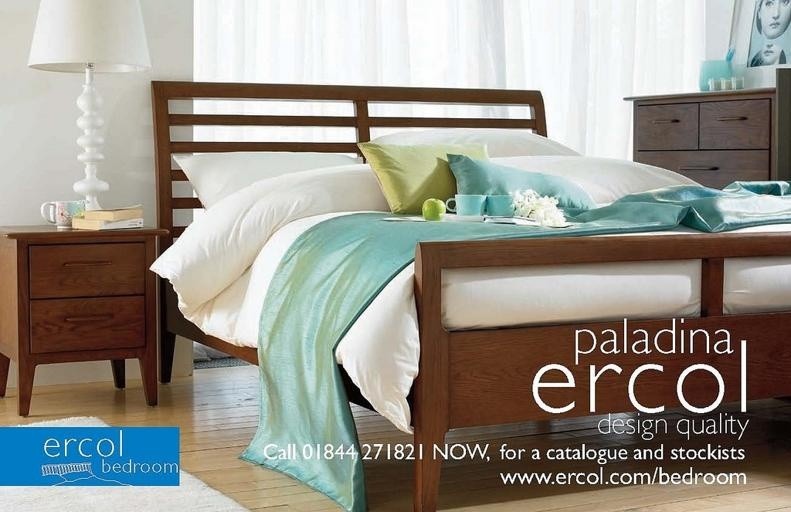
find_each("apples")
[422,198,446,221]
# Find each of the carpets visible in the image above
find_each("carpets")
[1,416,250,512]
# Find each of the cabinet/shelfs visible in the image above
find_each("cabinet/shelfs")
[622,68,791,191]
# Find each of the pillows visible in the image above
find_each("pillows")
[171,126,705,216]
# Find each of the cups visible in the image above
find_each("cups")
[709,76,743,90]
[40,200,87,228]
[443,193,515,220]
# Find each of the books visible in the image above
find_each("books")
[72,215,144,230]
[85,205,144,222]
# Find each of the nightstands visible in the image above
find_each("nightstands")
[1,224,170,417]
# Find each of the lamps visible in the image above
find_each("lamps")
[27,0,153,212]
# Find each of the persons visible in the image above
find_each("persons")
[760,42,782,65]
[755,0,791,39]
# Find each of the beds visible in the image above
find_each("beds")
[151,79,791,511]
